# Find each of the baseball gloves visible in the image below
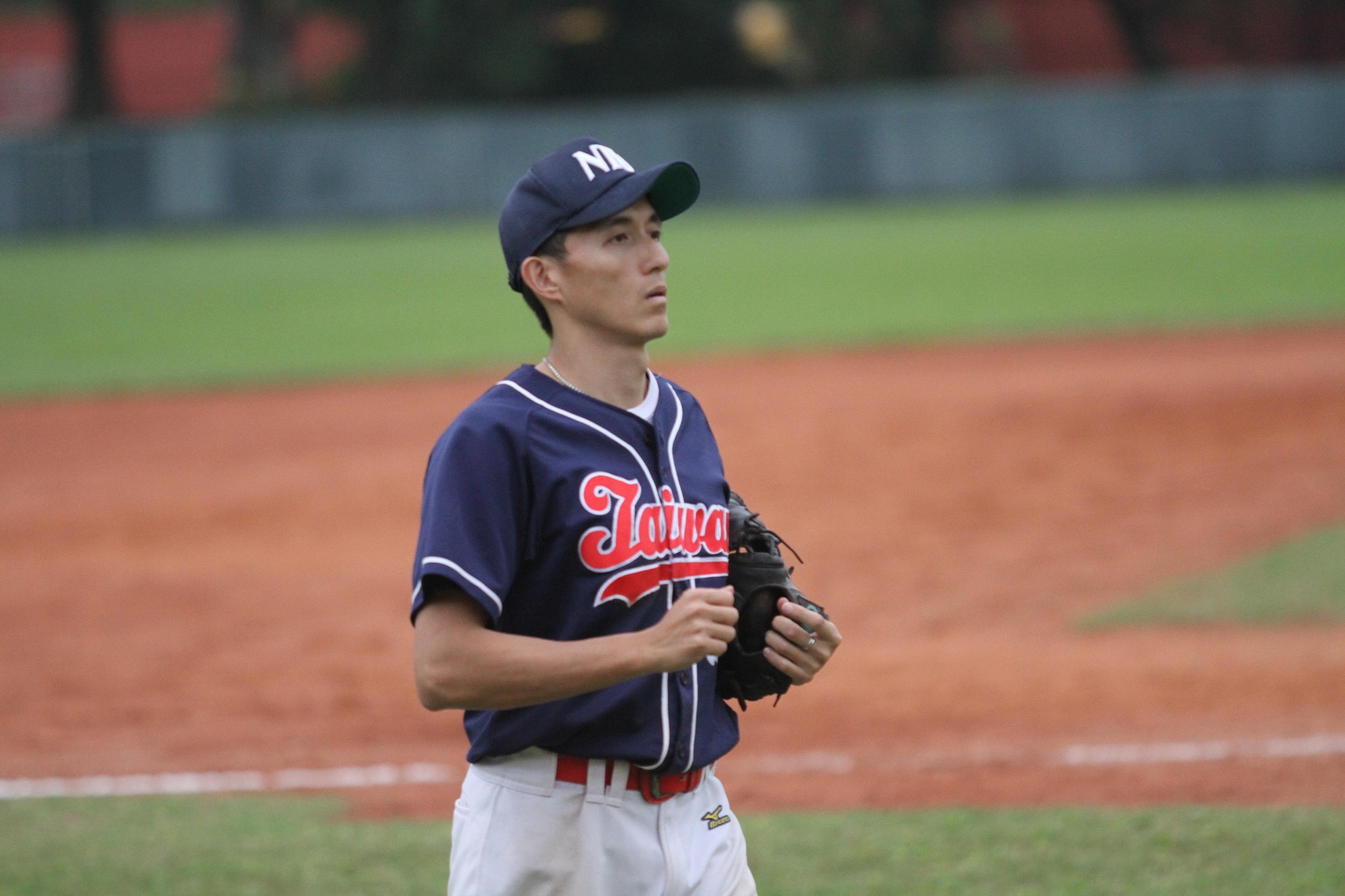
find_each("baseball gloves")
[713,489,828,715]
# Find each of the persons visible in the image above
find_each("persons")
[408,136,844,896]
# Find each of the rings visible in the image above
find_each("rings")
[801,633,815,651]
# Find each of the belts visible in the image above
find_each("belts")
[556,755,704,806]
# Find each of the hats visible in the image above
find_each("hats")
[498,137,697,270]
